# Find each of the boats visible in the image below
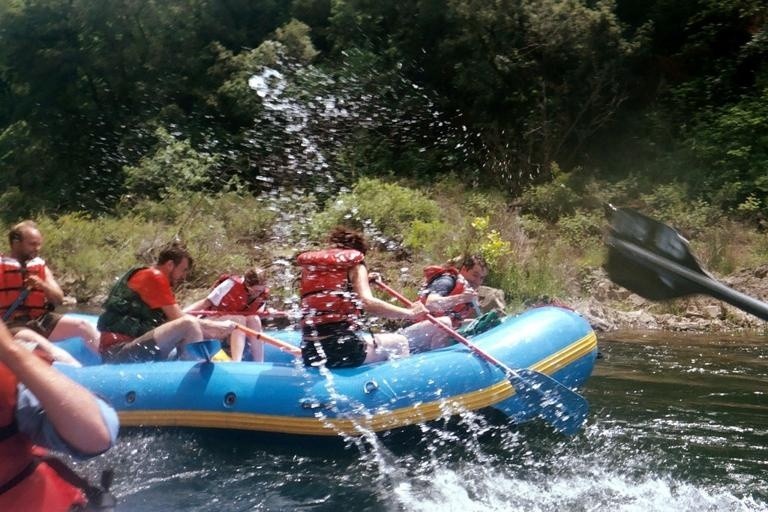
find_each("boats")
[15,303,600,458]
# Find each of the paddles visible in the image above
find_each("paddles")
[603,205,766,322]
[373,277,590,435]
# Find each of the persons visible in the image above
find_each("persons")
[0,316,121,512]
[97,238,236,365]
[183,267,279,363]
[0,220,100,368]
[395,254,506,354]
[294,220,430,368]
